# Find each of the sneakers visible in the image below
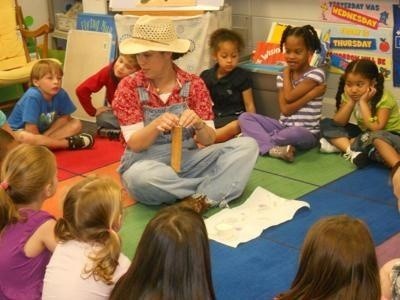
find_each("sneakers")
[346,147,369,167]
[170,193,210,216]
[275,143,296,162]
[62,133,94,150]
[318,137,342,155]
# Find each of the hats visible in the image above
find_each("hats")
[117,14,191,57]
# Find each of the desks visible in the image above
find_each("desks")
[51,29,68,49]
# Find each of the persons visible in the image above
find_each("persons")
[238,25,327,162]
[0,110,63,300]
[110,206,216,300]
[8,60,94,150]
[319,60,400,168]
[379,258,400,300]
[199,28,257,149]
[76,53,142,139]
[392,166,400,211]
[372,131,400,169]
[112,15,259,215]
[41,175,132,300]
[273,214,381,300]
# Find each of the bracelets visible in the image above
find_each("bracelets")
[192,122,206,130]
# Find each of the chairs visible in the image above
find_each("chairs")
[0,0,50,109]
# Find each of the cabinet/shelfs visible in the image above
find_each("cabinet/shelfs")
[249,71,281,120]
